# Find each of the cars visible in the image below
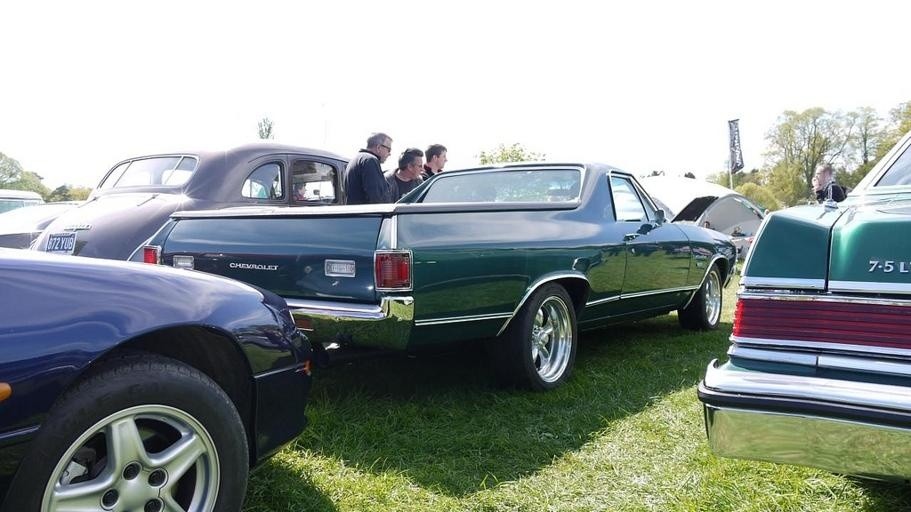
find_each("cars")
[696,130,910,486]
[1,246,315,512]
[0,187,85,248]
[32,141,350,262]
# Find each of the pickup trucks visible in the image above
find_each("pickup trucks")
[145,159,740,391]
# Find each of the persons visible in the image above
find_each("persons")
[342,131,394,202]
[811,162,848,204]
[418,143,448,181]
[382,147,425,202]
[292,176,309,201]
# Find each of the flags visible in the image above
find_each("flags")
[727,116,745,176]
